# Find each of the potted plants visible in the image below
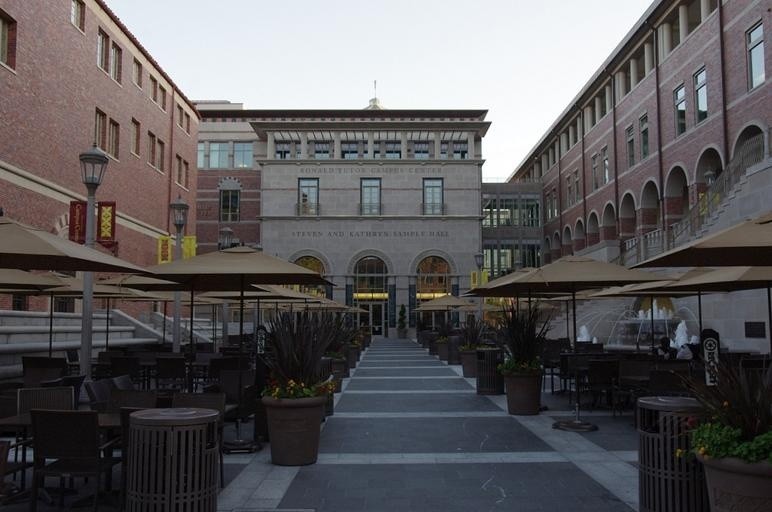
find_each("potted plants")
[677,346,772,512]
[317,321,373,392]
[258,302,343,465]
[396,304,408,338]
[416,319,481,376]
[494,289,553,415]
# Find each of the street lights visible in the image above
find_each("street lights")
[169,193,190,355]
[219,226,234,349]
[251,243,262,342]
[72,142,108,405]
[474,251,485,334]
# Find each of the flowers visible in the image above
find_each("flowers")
[262,378,337,400]
[678,416,772,466]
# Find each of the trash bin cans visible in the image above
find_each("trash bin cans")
[447,335,463,364]
[636,397,708,512]
[477,348,505,395]
[349,345,356,368]
[321,357,334,422]
[127,408,220,512]
[429,332,440,355]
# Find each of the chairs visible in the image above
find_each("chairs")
[483,328,771,432]
